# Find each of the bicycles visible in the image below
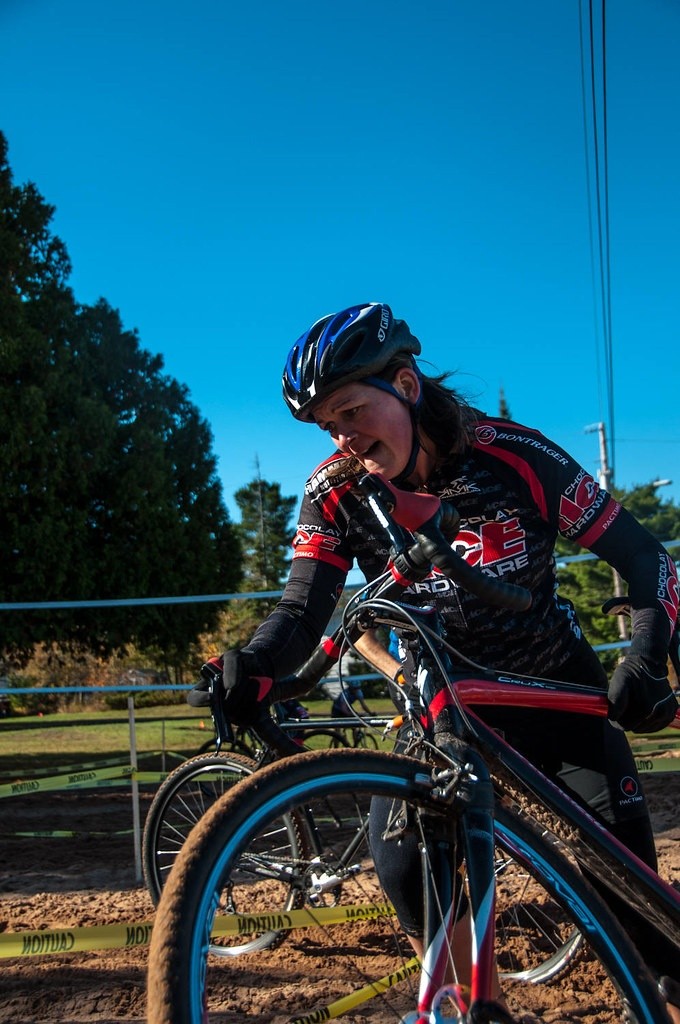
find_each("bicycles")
[142,473,680,1024]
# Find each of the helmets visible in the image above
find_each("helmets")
[282,303,421,421]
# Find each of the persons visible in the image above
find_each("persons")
[198,304,680,1024]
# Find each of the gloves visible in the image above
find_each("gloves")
[184,652,260,726]
[608,657,678,735]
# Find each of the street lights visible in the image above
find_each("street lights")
[583,421,627,641]
